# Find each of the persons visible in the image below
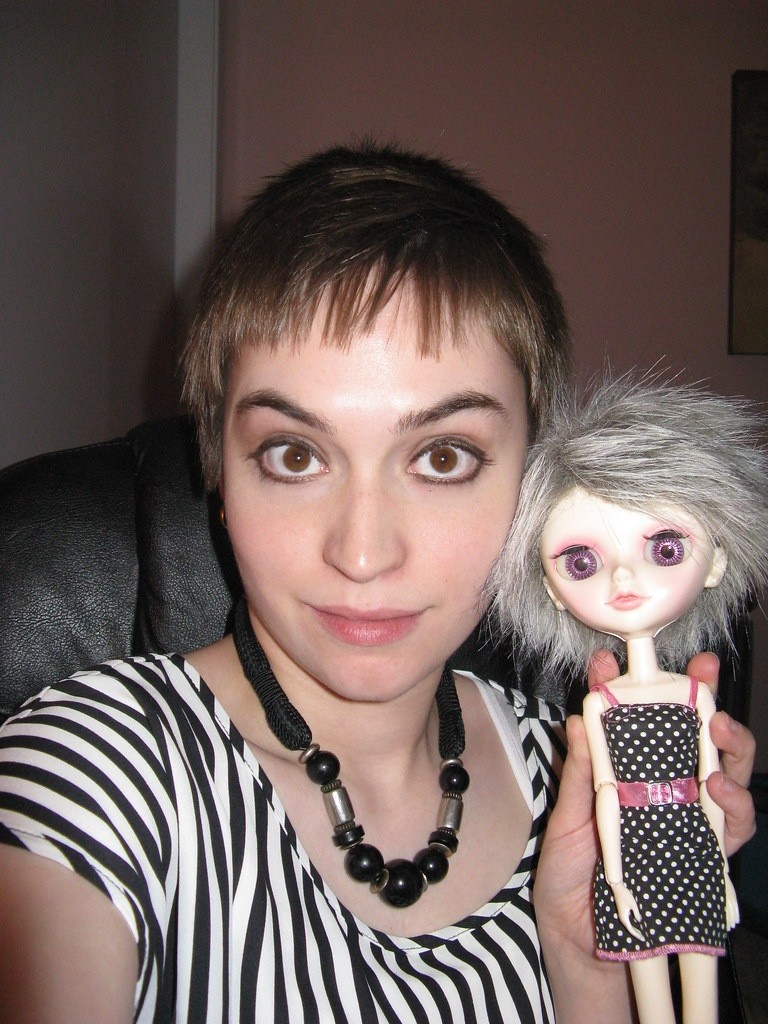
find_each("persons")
[489,357,765,1024]
[1,147,755,1021]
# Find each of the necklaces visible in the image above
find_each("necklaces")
[225,594,468,906]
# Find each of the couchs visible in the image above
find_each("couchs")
[1,416,768,1024]
